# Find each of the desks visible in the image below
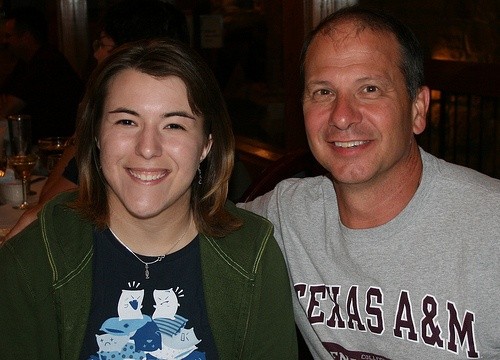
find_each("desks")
[0,174,79,247]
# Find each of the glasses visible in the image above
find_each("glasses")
[94,40,114,52]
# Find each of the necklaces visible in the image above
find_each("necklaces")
[107,220,191,280]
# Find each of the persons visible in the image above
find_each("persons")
[237,4,500,360]
[0,40,298,360]
[0,0,189,245]
